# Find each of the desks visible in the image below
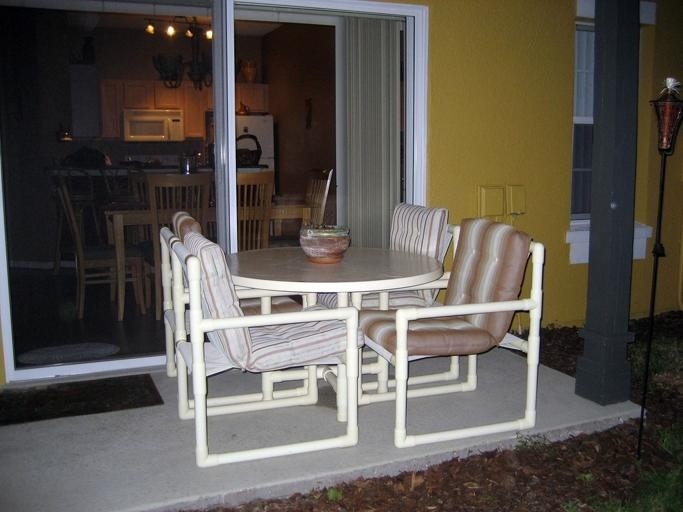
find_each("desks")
[225,247,443,420]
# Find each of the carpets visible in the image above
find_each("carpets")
[0,348,647,512]
[17,344,120,364]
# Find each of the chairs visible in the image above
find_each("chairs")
[358,220,545,447]
[158,208,316,407]
[170,234,365,467]
[56,167,334,323]
[315,200,452,399]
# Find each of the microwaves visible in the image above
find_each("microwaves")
[122,109,184,143]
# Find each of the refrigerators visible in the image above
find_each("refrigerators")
[210,112,276,177]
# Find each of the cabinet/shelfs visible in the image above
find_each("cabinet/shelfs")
[98,79,270,138]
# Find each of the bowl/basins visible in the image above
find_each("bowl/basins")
[178,155,201,173]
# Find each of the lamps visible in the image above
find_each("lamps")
[145,17,241,90]
[59,128,76,140]
